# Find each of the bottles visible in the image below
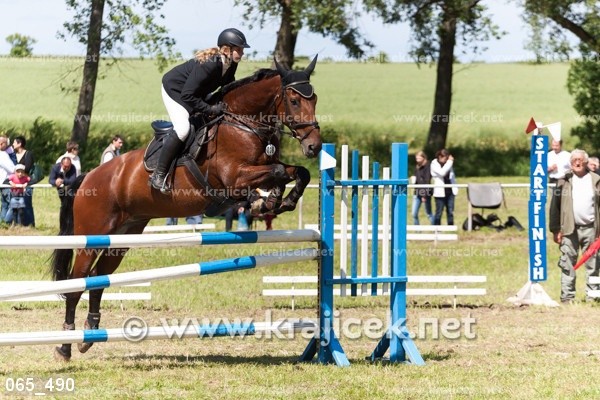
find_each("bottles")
[15,163,26,171]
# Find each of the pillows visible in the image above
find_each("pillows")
[217,28,250,48]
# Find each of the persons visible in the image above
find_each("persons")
[223,199,252,231]
[587,157,600,175]
[56,141,82,177]
[49,157,76,203]
[411,151,435,225]
[100,134,124,166]
[0,136,17,223]
[6,138,36,227]
[430,148,458,225]
[4,164,32,226]
[148,28,250,190]
[166,214,203,225]
[549,149,600,302]
[547,139,571,183]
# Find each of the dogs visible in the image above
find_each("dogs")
[47,54,323,362]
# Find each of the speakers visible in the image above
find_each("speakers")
[147,129,184,195]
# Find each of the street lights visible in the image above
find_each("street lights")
[25,150,44,185]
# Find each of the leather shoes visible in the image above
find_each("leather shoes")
[211,101,226,116]
